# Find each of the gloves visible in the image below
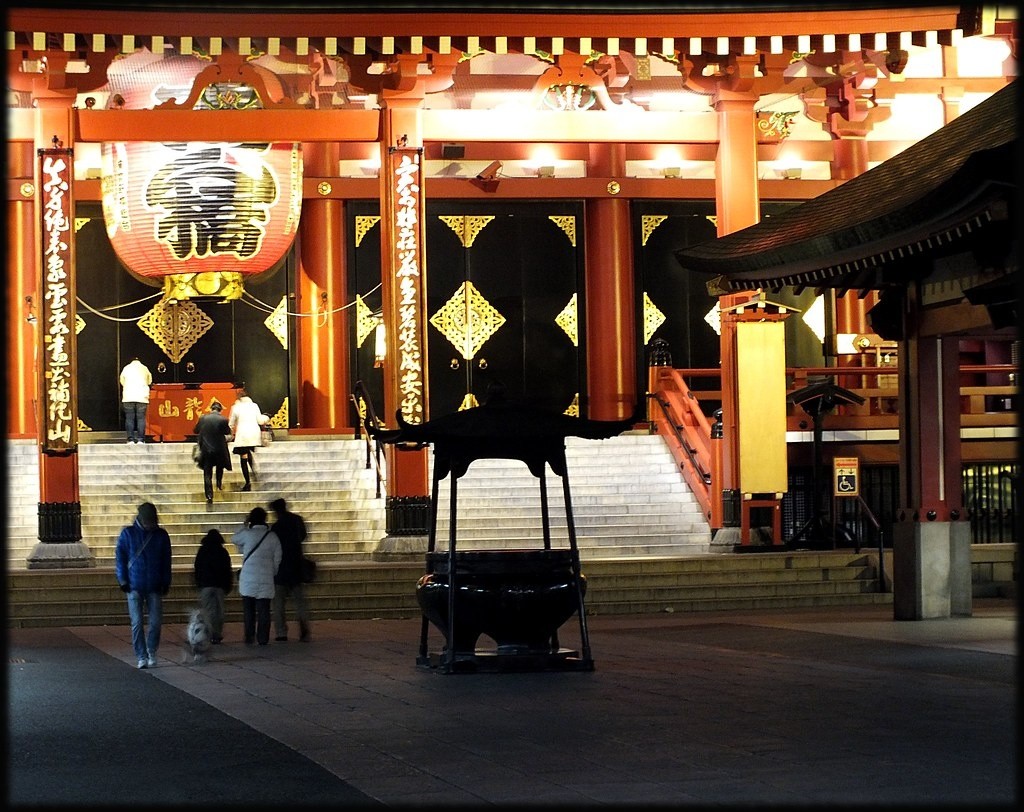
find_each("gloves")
[120,584,130,593]
[159,584,169,596]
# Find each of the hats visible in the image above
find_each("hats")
[137,502,157,521]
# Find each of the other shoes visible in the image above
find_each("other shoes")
[253,474,258,482]
[275,636,288,642]
[210,632,223,644]
[137,438,145,444]
[207,492,213,503]
[258,637,269,645]
[241,483,251,491]
[300,630,310,642]
[245,635,256,644]
[216,480,223,490]
[126,439,135,444]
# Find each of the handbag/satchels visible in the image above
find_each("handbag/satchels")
[236,568,241,580]
[192,445,201,461]
[302,556,317,584]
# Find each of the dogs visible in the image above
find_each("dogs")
[184,608,214,664]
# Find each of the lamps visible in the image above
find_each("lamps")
[537,165,555,178]
[783,168,803,180]
[84,167,101,179]
[663,167,681,180]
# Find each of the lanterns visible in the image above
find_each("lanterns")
[98,52,305,305]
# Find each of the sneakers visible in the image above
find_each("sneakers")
[147,652,157,666]
[137,659,149,669]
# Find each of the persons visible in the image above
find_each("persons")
[194,528,234,645]
[231,498,312,647]
[228,389,269,492]
[193,401,231,506]
[120,356,152,445]
[115,501,173,667]
